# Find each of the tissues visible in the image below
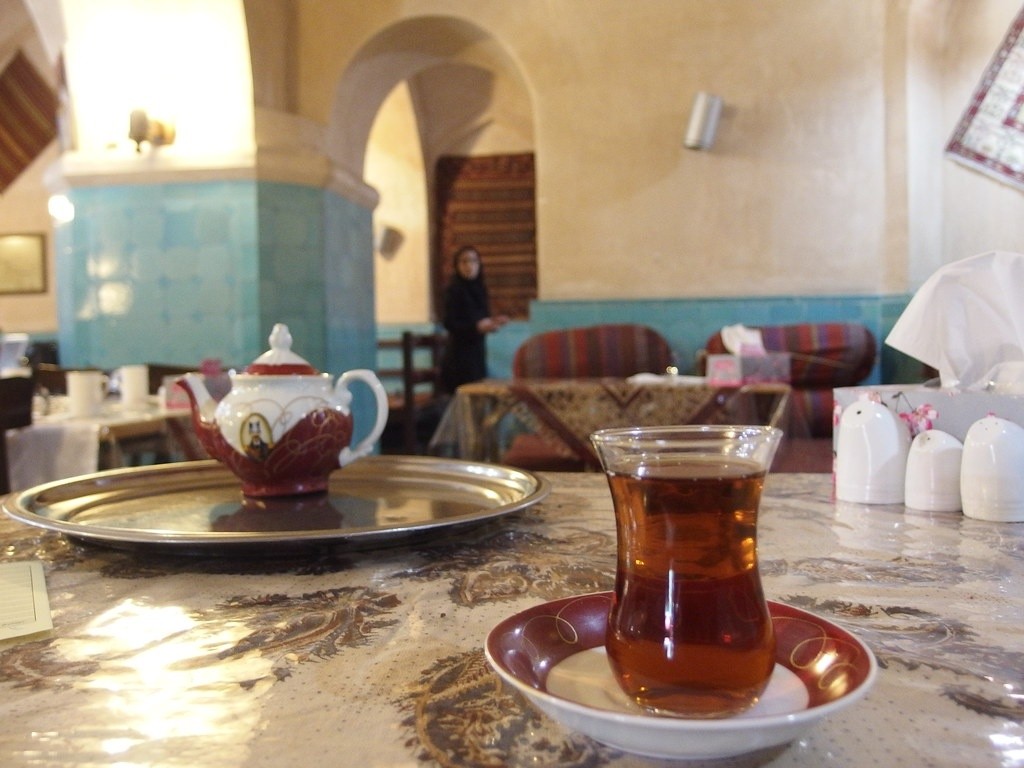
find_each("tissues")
[830,250,1024,481]
[705,323,793,386]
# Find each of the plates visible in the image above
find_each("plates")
[485,591,878,759]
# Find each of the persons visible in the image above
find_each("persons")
[438,246,510,404]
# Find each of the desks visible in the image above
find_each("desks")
[0,473,1024,768]
[445,379,789,473]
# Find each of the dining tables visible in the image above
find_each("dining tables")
[7,394,197,489]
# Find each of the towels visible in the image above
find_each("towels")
[5,421,128,491]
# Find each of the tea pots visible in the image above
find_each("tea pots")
[174,323,389,499]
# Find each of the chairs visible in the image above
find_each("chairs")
[500,324,675,468]
[700,319,878,468]
[363,332,443,460]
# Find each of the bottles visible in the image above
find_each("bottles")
[834,399,1024,521]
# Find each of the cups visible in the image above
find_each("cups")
[588,424,782,719]
[113,365,148,409]
[66,370,110,413]
